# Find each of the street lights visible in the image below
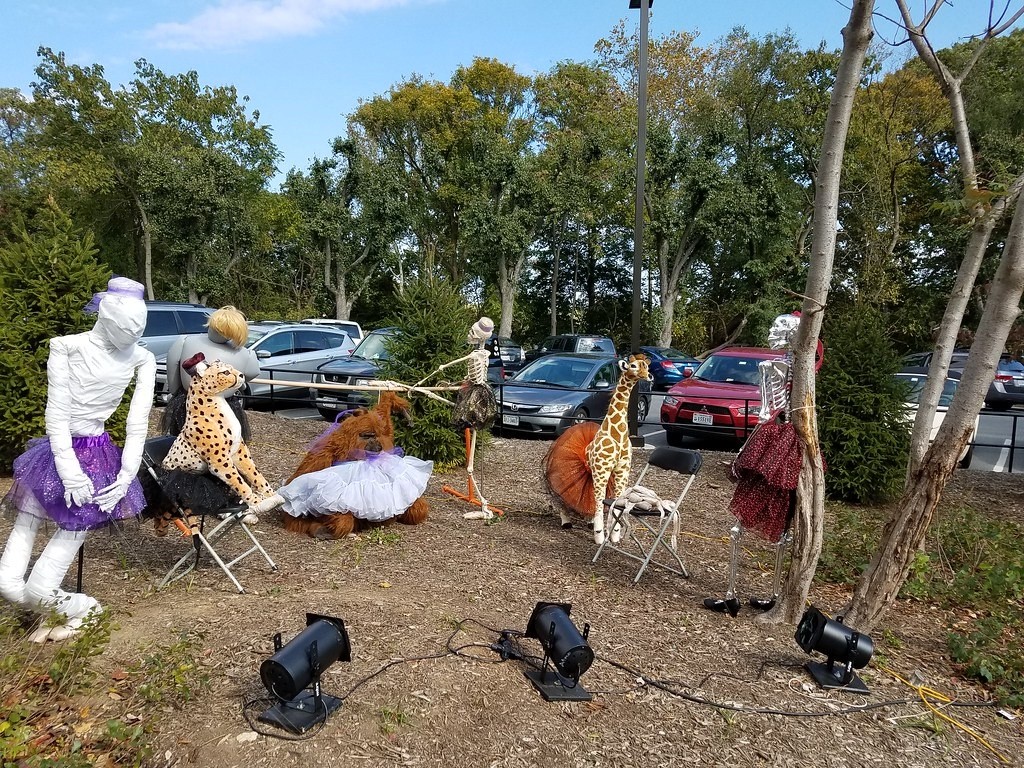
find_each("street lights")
[626,0,660,360]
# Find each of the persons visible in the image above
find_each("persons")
[157,306,259,443]
[1,276,158,643]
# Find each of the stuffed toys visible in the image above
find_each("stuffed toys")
[539,353,655,544]
[282,394,428,540]
[152,352,283,537]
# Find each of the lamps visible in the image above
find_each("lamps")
[795,604,874,698]
[525,601,594,704]
[253,612,357,734]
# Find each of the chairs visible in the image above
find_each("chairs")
[591,444,704,583]
[135,433,279,594]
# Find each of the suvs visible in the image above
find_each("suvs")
[897,350,1024,414]
[527,333,618,364]
[124,298,227,397]
[309,326,506,433]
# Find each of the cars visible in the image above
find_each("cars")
[494,337,527,379]
[228,325,358,410]
[660,347,797,450]
[489,353,652,440]
[883,372,988,471]
[625,346,704,391]
[300,318,366,352]
